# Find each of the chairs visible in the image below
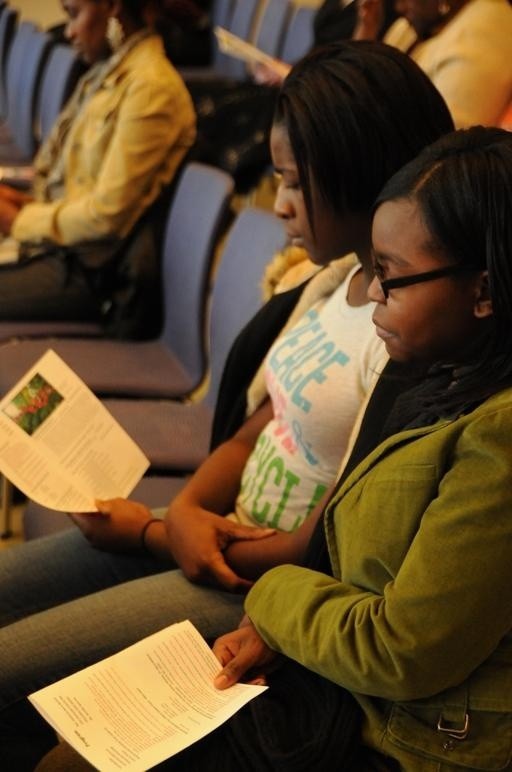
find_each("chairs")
[1,0,316,164]
[2,190,166,340]
[101,208,294,471]
[23,476,198,547]
[0,161,234,402]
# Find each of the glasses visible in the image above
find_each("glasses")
[375,261,466,298]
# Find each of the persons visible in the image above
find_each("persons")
[1,0,512,771]
